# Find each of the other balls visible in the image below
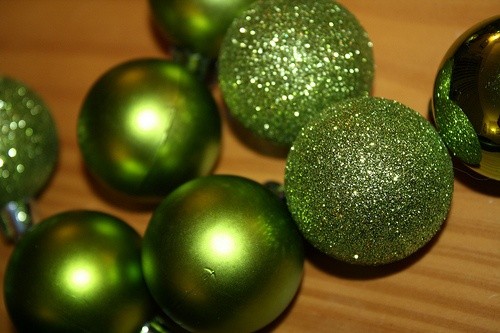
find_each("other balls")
[6,208,151,333]
[74,58,224,204]
[142,173,305,333]
[436,57,453,99]
[219,0,376,145]
[0,76,60,243]
[146,0,244,82]
[283,96,455,266]
[434,95,484,167]
